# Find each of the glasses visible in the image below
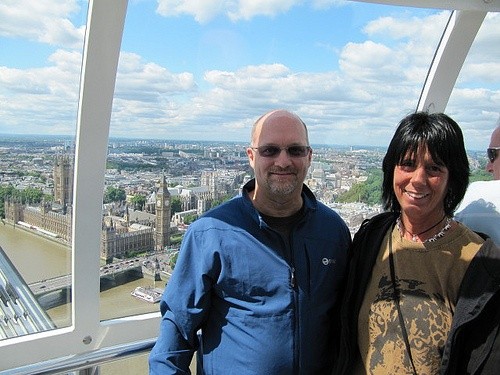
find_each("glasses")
[251,146,311,159]
[486,147,500,163]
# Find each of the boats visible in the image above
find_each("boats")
[130,285,165,304]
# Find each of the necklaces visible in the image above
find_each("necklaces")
[396,215,451,244]
[395,214,447,242]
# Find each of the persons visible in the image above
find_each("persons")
[148,110,352,375]
[329,112,500,375]
[486,126,499,179]
